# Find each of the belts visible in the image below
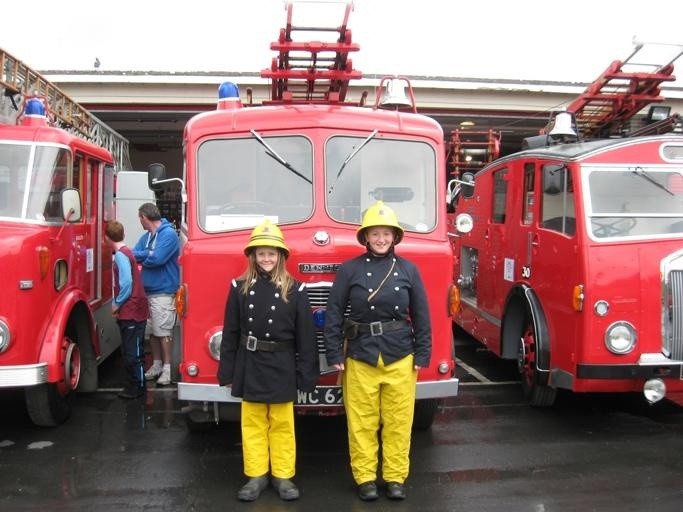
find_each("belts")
[357,320,406,336]
[240,335,296,353]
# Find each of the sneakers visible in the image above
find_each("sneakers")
[237,476,269,501]
[386,482,407,499]
[118,382,144,399]
[359,481,379,500]
[271,478,300,500]
[157,367,170,386]
[144,366,162,380]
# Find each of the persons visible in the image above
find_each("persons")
[215,218,319,503]
[130,202,181,386]
[322,199,432,501]
[104,219,150,399]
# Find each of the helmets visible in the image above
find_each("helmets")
[356,201,404,245]
[244,219,289,259]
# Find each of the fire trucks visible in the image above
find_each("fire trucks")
[445,34,681,417]
[147,3,475,433]
[0,49,157,425]
